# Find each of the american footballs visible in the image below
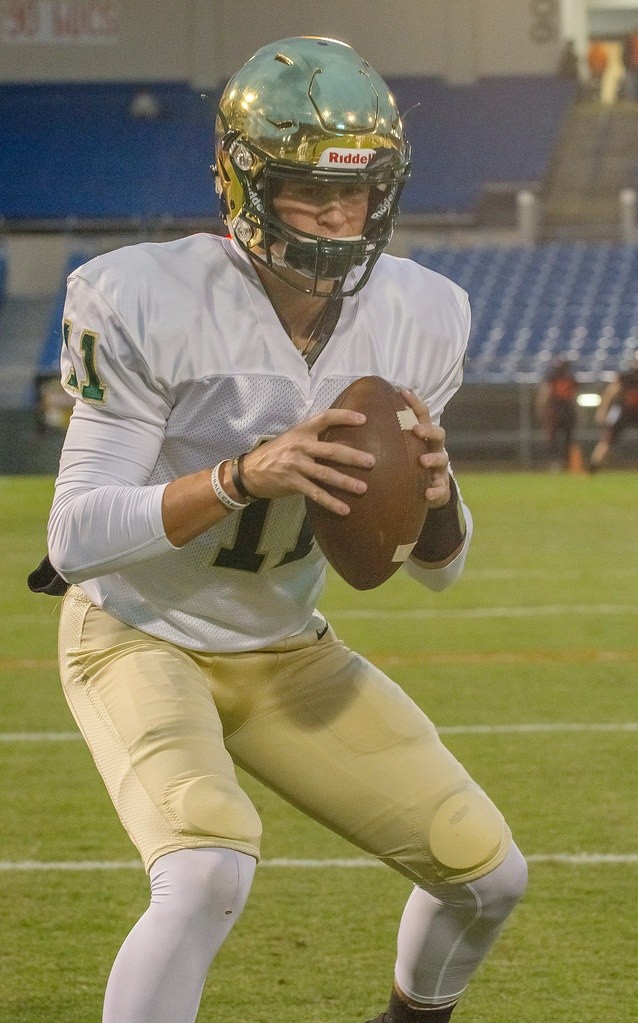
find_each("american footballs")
[302,374,435,594]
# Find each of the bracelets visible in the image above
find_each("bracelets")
[211,459,250,511]
[231,453,260,503]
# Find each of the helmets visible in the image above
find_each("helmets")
[210,37,415,281]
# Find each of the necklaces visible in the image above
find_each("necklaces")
[291,305,327,354]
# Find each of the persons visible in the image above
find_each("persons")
[47,37,529,1023]
[556,30,638,103]
[536,348,638,474]
